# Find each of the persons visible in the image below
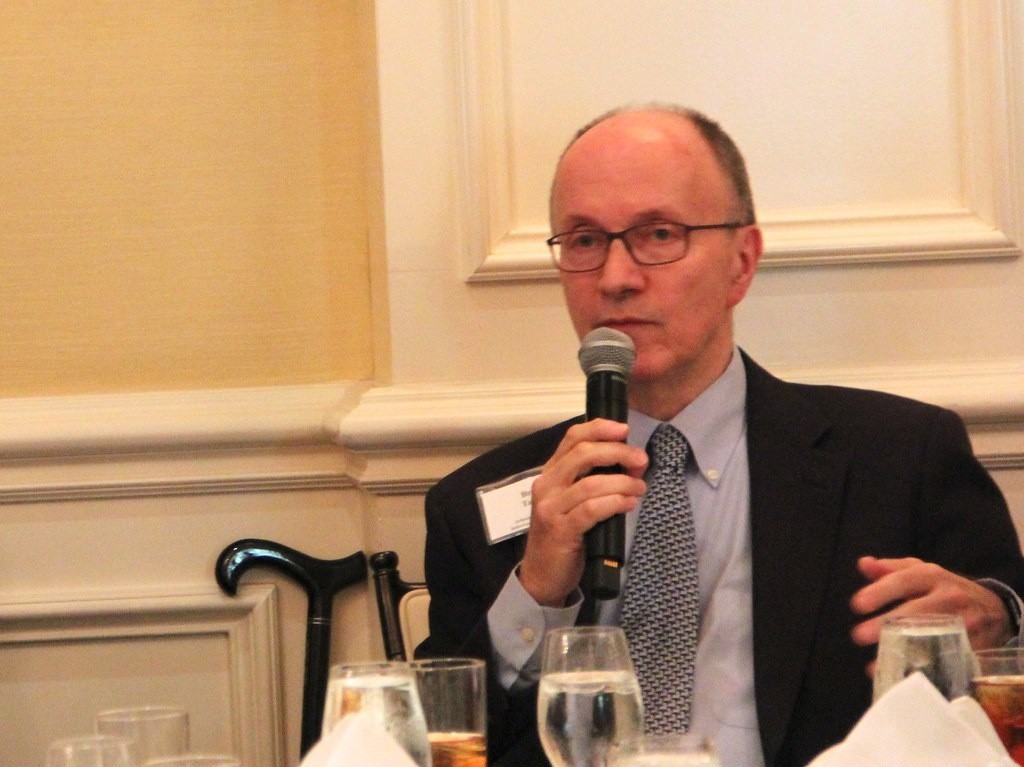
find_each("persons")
[414,104,1024,767]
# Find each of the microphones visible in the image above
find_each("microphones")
[576,326,638,600]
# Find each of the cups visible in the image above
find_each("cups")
[46,735,147,767]
[971,648,1024,767]
[145,754,242,767]
[320,661,433,767]
[94,707,192,763]
[407,658,488,767]
[614,736,722,767]
[872,613,984,709]
[536,625,644,767]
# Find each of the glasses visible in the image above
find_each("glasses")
[547,220,743,273]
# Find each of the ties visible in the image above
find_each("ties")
[617,421,700,736]
